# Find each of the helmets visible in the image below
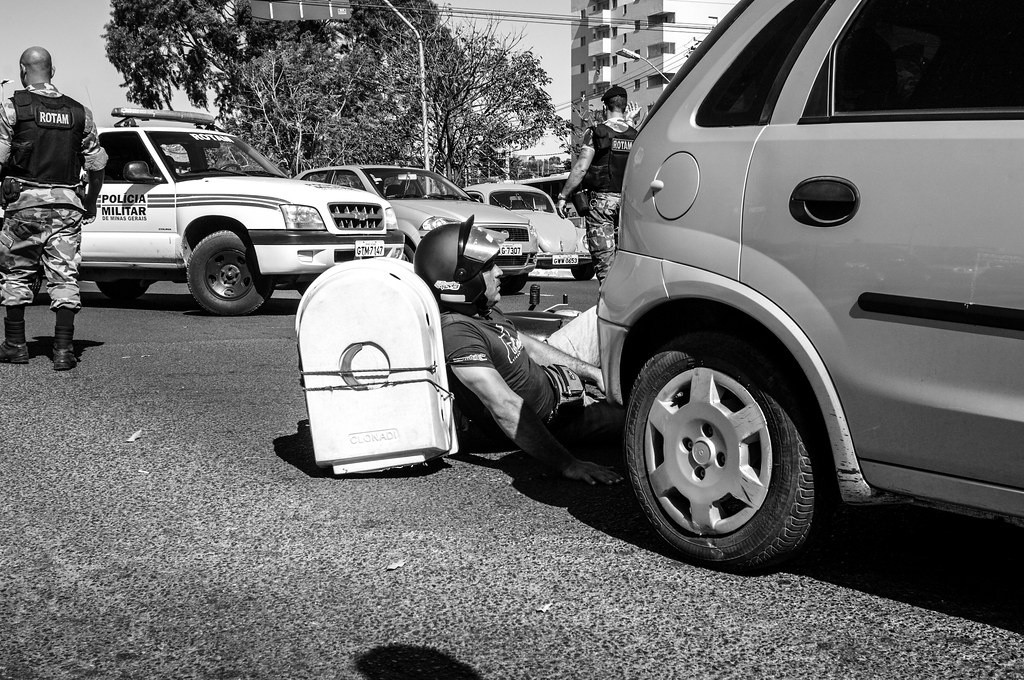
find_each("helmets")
[414,213,501,314]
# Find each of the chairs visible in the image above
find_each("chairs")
[144,156,176,175]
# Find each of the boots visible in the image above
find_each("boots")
[52,325,77,369]
[0,317,29,363]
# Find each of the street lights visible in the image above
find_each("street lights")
[0,79,13,103]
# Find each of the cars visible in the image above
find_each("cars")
[461,183,597,281]
[290,165,539,296]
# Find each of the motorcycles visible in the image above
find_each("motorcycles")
[296,258,585,478]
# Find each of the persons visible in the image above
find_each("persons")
[413,214,627,484]
[0,46,109,369]
[554,86,639,284]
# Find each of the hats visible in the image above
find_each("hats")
[600,86,627,100]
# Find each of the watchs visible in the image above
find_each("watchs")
[558,192,567,199]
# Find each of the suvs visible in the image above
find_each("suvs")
[0,107,405,315]
[597,0,1024,571]
